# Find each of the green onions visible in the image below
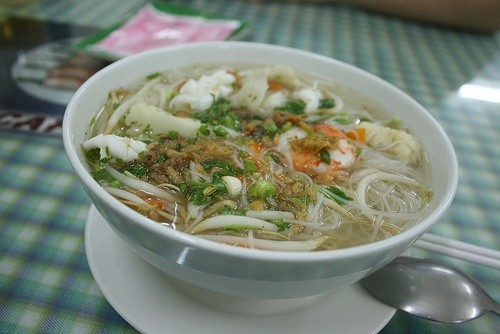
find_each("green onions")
[83,95,372,234]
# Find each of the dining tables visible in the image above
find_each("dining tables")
[0,0,500,334]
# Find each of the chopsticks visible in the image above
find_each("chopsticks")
[413,232,500,270]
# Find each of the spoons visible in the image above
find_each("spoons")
[361,254,500,324]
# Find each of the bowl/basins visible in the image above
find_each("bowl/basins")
[62,40,458,299]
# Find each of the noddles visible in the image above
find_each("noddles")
[81,62,433,253]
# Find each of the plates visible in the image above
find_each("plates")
[84,202,413,334]
